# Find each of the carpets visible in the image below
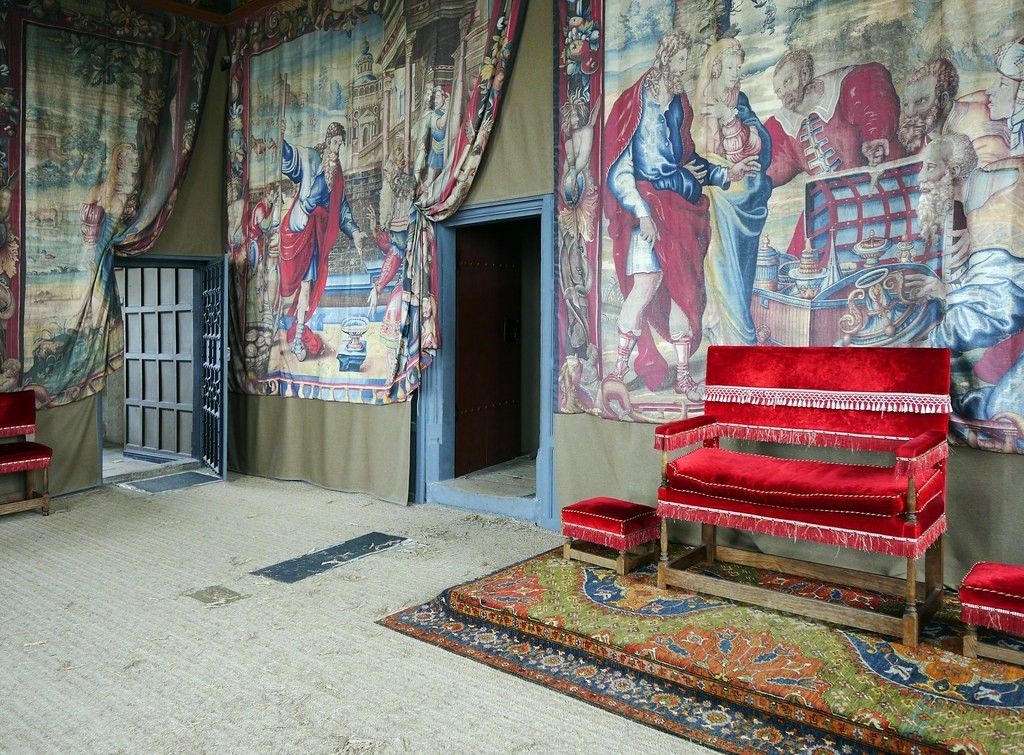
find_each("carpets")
[373,539,1024,755]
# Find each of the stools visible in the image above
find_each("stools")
[560,497,662,577]
[956,560,1024,667]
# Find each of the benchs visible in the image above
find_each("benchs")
[654,344,953,649]
[0,390,54,517]
